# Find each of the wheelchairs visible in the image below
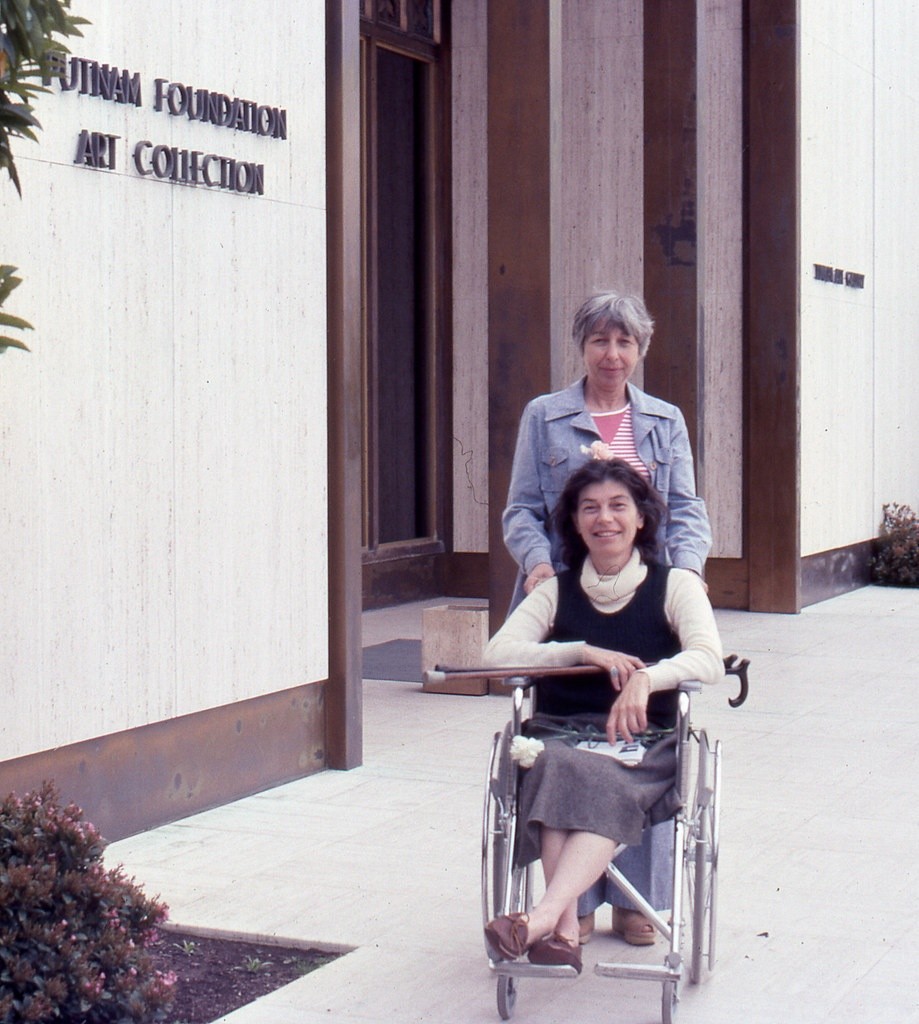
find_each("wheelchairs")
[475,576,722,1023]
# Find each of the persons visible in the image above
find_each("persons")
[481,456,727,973]
[501,292,713,948]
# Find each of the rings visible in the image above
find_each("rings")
[610,665,619,677]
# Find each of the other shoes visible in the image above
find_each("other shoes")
[578,910,594,942]
[528,933,582,974]
[482,912,534,963]
[611,905,656,947]
